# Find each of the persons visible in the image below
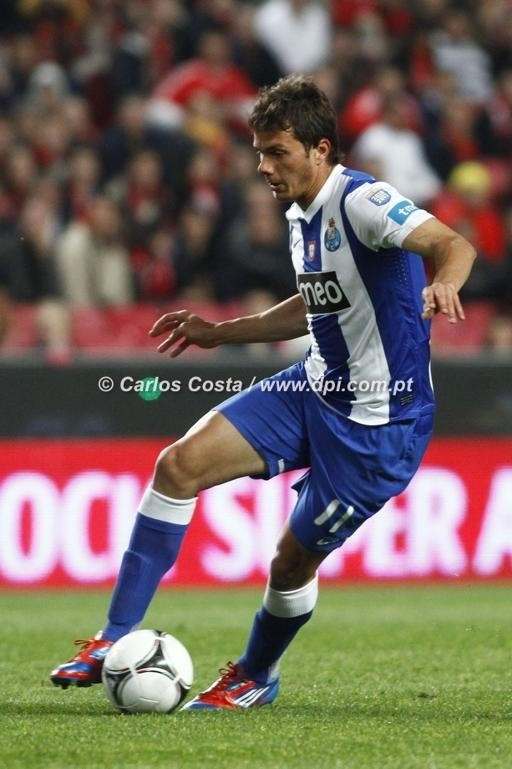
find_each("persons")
[2,1,512,362]
[50,76,480,713]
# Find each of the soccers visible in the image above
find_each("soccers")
[101,629,195,714]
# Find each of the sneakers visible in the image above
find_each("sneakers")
[50,631,114,689]
[178,665,279,710]
